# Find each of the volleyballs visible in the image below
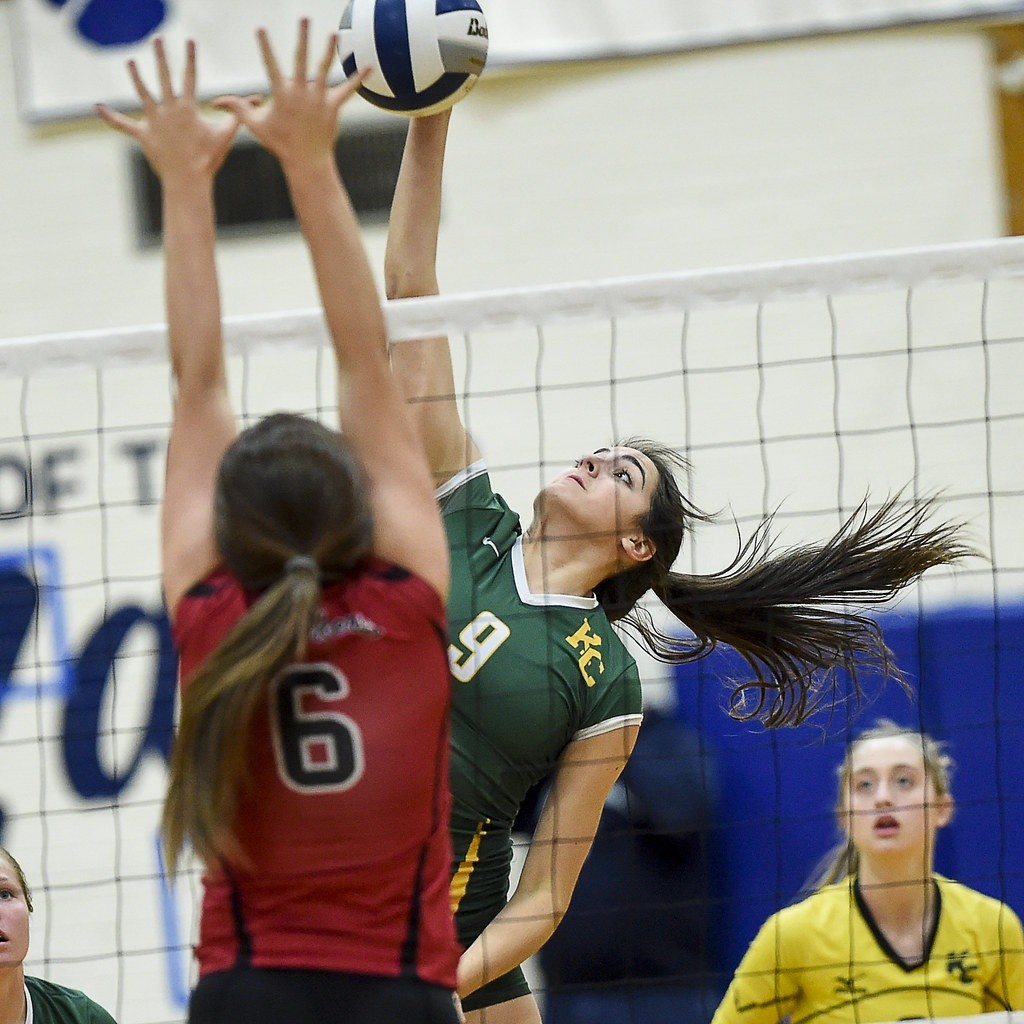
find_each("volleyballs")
[334,0,489,119]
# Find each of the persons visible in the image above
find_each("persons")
[0,845,118,1024]
[708,716,1024,1024]
[91,18,465,1024]
[381,108,996,1024]
